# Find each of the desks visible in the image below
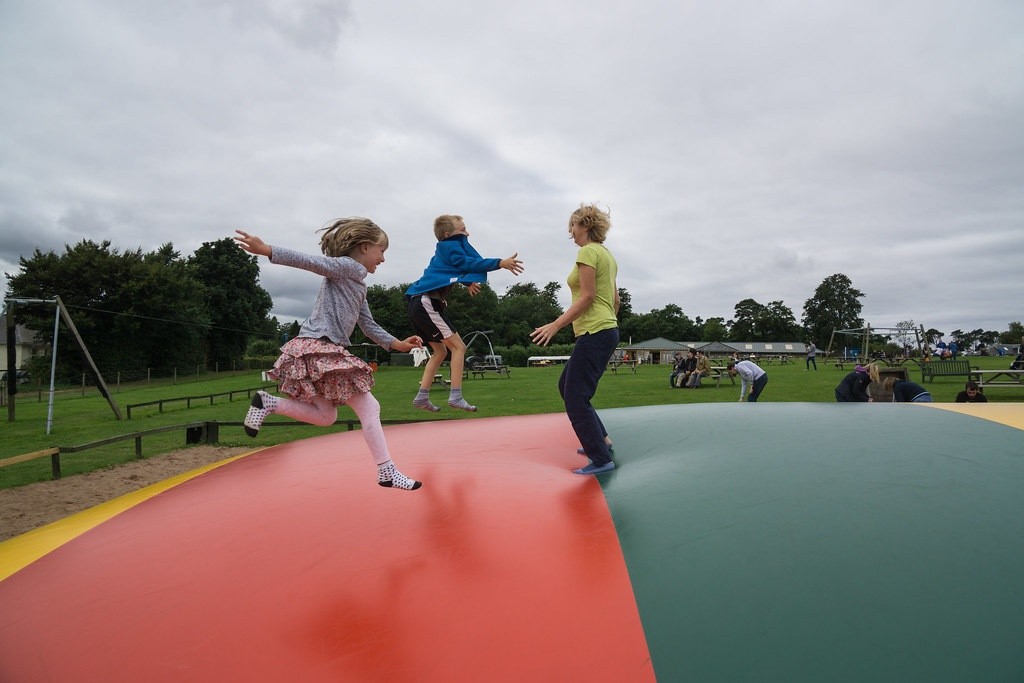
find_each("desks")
[708,358,723,366]
[835,357,861,371]
[895,357,921,368]
[767,354,788,366]
[473,365,509,380]
[970,369,1024,394]
[738,356,761,365]
[609,359,638,375]
[433,374,449,391]
[710,367,736,387]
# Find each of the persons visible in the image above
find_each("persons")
[623,354,629,360]
[871,349,908,358]
[834,363,881,402]
[233,216,423,491]
[923,340,958,361]
[405,215,524,412]
[883,376,934,402]
[727,360,768,402]
[806,339,817,372]
[529,200,621,474]
[732,351,759,359]
[668,348,710,389]
[955,382,988,403]
[1009,336,1024,370]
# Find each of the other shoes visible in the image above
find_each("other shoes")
[692,386,698,389]
[688,386,694,389]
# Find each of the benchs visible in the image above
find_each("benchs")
[974,380,1024,388]
[832,362,866,366]
[472,371,486,379]
[886,361,920,366]
[610,366,637,370]
[444,380,451,384]
[920,360,981,385]
[707,372,737,378]
[752,359,790,362]
